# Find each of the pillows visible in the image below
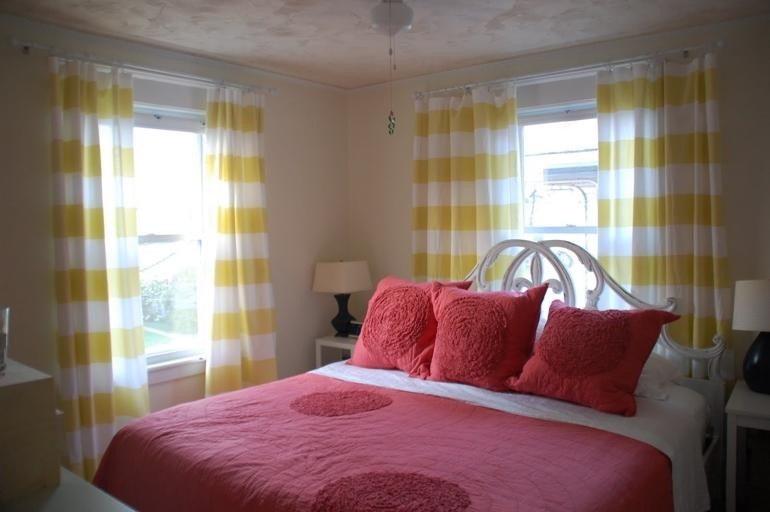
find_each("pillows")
[430,280,549,392]
[346,274,473,379]
[509,299,682,415]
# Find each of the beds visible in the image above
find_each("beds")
[116,239,725,512]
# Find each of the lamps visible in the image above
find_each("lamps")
[369,0,415,135]
[731,280,770,394]
[310,260,372,337]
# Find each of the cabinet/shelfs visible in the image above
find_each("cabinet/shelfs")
[0,356,139,512]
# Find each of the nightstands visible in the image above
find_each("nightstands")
[724,379,770,512]
[315,331,356,368]
